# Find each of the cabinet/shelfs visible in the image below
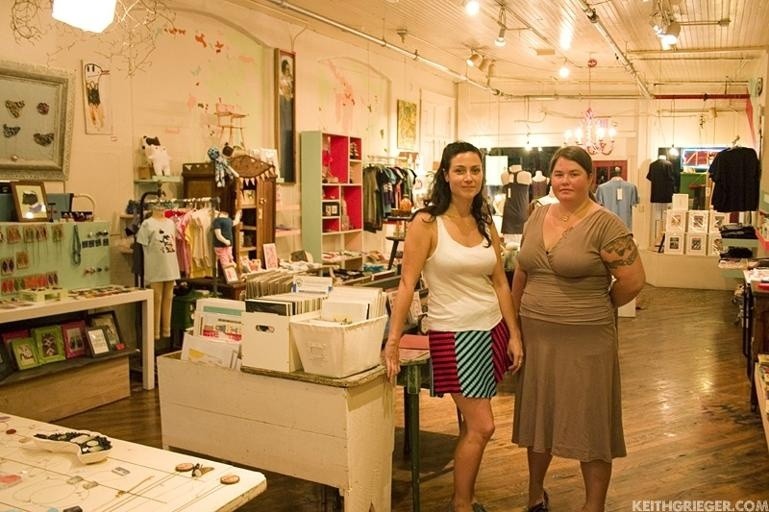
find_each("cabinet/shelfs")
[181,161,278,308]
[299,129,365,273]
[744,267,769,411]
[719,236,760,279]
[386,215,412,270]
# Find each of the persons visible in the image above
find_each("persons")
[511,146,645,512]
[501,164,532,234]
[647,154,673,202]
[213,209,243,269]
[137,206,182,340]
[381,141,524,512]
[532,170,551,200]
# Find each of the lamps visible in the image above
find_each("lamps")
[465,46,497,79]
[649,0,683,52]
[564,54,619,155]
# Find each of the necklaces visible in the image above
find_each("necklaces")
[559,202,589,221]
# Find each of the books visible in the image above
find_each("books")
[244,269,423,335]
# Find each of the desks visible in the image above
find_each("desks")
[1,411,266,512]
[752,366,769,452]
[156,347,394,511]
[391,350,438,511]
[0,283,155,398]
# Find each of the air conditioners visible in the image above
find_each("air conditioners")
[494,23,509,48]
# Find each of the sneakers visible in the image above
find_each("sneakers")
[529,489,550,511]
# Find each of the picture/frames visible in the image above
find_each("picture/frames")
[0,310,128,376]
[10,181,51,222]
[272,48,298,186]
[663,210,730,257]
[0,58,78,180]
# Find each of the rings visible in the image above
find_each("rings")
[518,361,522,365]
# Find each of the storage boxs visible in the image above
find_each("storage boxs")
[239,310,320,375]
[288,314,391,376]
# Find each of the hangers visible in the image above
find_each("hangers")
[365,156,411,170]
[152,197,227,215]
[726,137,744,151]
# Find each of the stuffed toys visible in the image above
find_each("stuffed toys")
[142,135,173,176]
